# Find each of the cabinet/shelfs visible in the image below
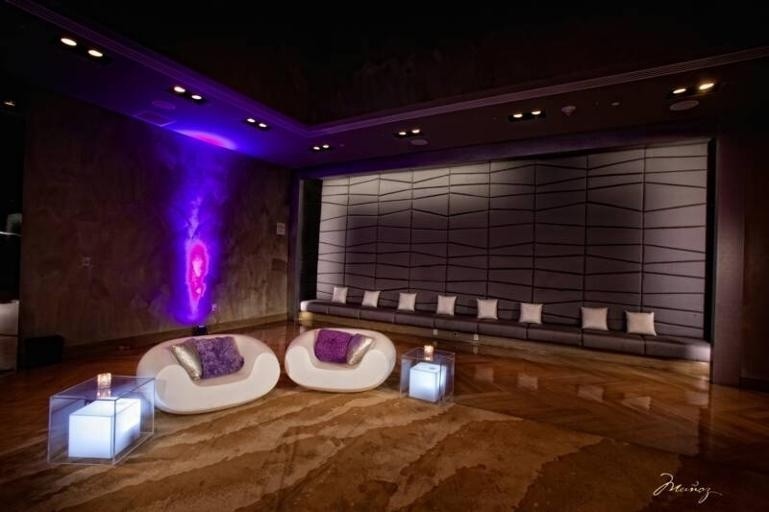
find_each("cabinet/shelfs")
[402,348,455,405]
[47,375,154,467]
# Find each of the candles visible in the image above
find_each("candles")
[424,344,434,356]
[96,373,112,389]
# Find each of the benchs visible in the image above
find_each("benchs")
[300,296,711,374]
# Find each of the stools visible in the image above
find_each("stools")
[136,334,283,415]
[284,327,397,395]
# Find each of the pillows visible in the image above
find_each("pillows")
[518,301,544,326]
[192,337,243,379]
[345,333,376,365]
[476,296,499,320]
[397,292,417,313]
[624,309,658,336]
[171,338,201,380]
[315,330,352,364]
[436,293,456,317]
[361,290,381,309]
[332,285,348,305]
[580,305,608,332]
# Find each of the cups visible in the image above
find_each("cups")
[97,373,111,389]
[96,390,111,401]
[423,345,433,360]
